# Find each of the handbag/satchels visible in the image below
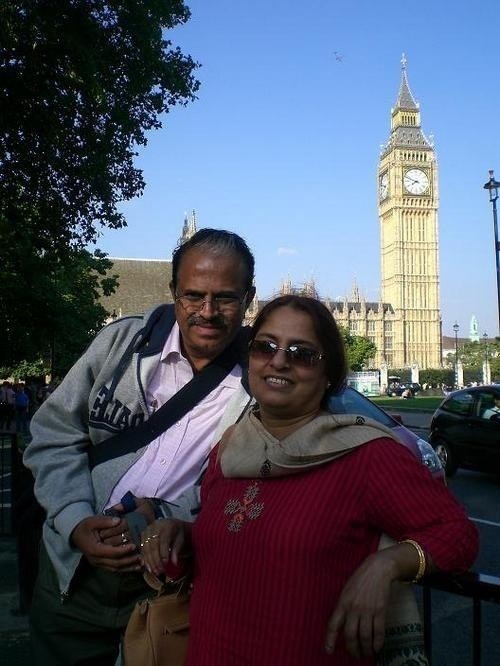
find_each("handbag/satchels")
[123,571,191,666]
[10,465,46,617]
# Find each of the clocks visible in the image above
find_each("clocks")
[380,173,389,200]
[402,167,429,194]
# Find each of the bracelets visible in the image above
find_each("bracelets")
[398,539,426,584]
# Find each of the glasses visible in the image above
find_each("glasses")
[247,339,330,365]
[175,290,249,312]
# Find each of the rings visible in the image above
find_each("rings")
[121,533,128,543]
[140,534,159,547]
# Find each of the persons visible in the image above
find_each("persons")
[482,396,500,419]
[22,228,256,666]
[140,295,479,666]
[0,378,29,433]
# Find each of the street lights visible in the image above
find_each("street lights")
[483,168,500,320]
[452,322,459,384]
[482,331,488,384]
[111,308,118,322]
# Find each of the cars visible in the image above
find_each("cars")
[329,381,500,489]
